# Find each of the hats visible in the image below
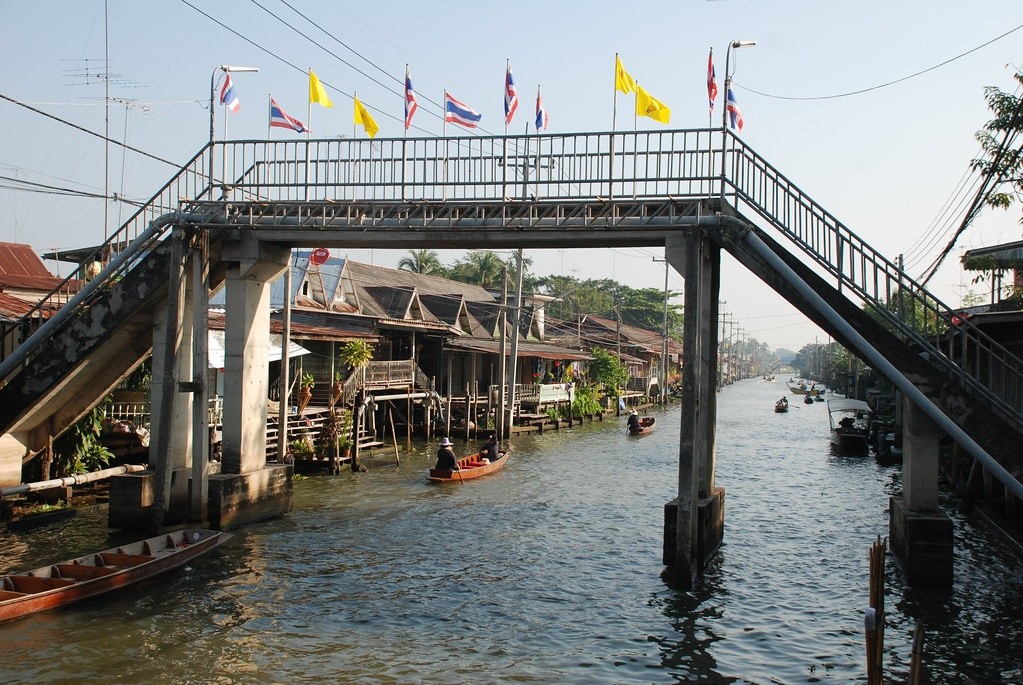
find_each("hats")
[438,437,453,445]
[631,410,638,415]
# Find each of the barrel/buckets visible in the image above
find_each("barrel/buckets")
[482,459,489,463]
[292,406,298,414]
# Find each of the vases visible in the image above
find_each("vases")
[535,380,540,384]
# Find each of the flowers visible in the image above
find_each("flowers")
[531,372,542,381]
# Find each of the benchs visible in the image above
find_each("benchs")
[470,462,487,466]
[462,465,476,469]
[639,422,653,426]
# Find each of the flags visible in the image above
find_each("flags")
[616,57,639,94]
[535,94,548,133]
[271,98,311,133]
[309,70,333,109]
[707,51,718,114]
[726,79,743,131]
[503,70,519,124]
[354,97,379,143]
[404,72,418,129]
[219,75,241,113]
[446,93,481,128]
[636,84,670,125]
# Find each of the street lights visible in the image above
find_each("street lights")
[209,64,258,200]
[720,40,757,196]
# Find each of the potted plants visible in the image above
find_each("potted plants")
[301,373,315,393]
[306,444,314,460]
[314,444,327,460]
[344,442,351,457]
[290,439,307,458]
[334,371,342,389]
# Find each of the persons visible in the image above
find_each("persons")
[804,392,820,399]
[431,437,460,479]
[627,407,642,432]
[798,379,815,391]
[778,395,788,406]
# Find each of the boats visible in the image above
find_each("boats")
[763,374,775,381]
[775,404,788,413]
[827,399,874,449]
[804,398,813,404]
[425,450,510,484]
[0,528,234,627]
[629,417,656,434]
[790,387,825,396]
[789,378,812,389]
[814,395,824,401]
